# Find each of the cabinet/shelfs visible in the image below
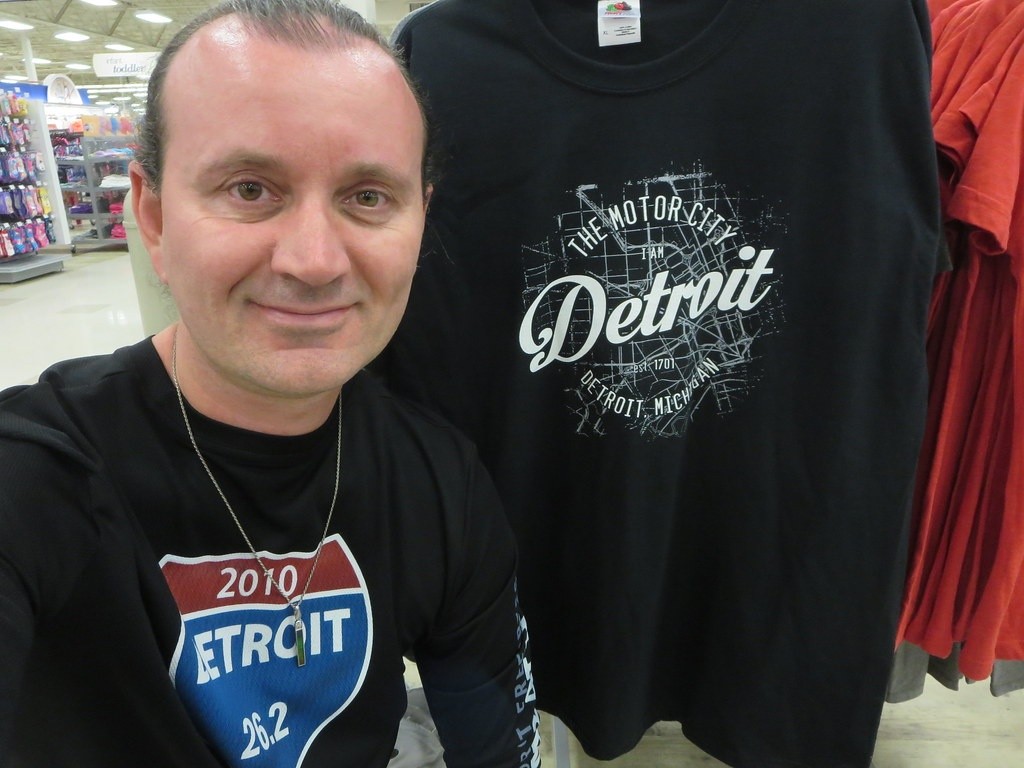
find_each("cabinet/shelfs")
[0,89,76,284]
[50,129,138,254]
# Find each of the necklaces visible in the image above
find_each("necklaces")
[172,325,343,668]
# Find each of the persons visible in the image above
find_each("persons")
[0,1,543,768]
